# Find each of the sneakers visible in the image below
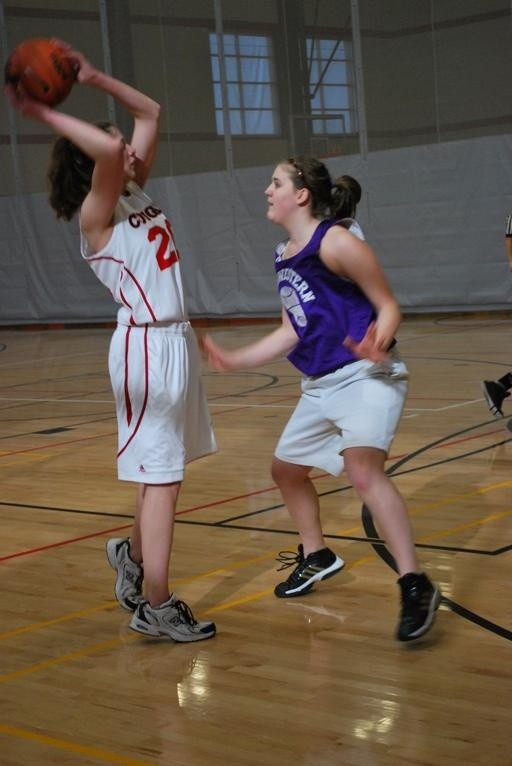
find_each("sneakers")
[106,537,143,612]
[128,592,215,643]
[397,571,441,641]
[481,380,511,419]
[274,543,345,598]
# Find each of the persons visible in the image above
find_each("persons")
[0,48,220,645]
[480,212,512,430]
[326,174,366,241]
[199,153,444,643]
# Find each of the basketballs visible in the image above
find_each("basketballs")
[7,38,74,108]
